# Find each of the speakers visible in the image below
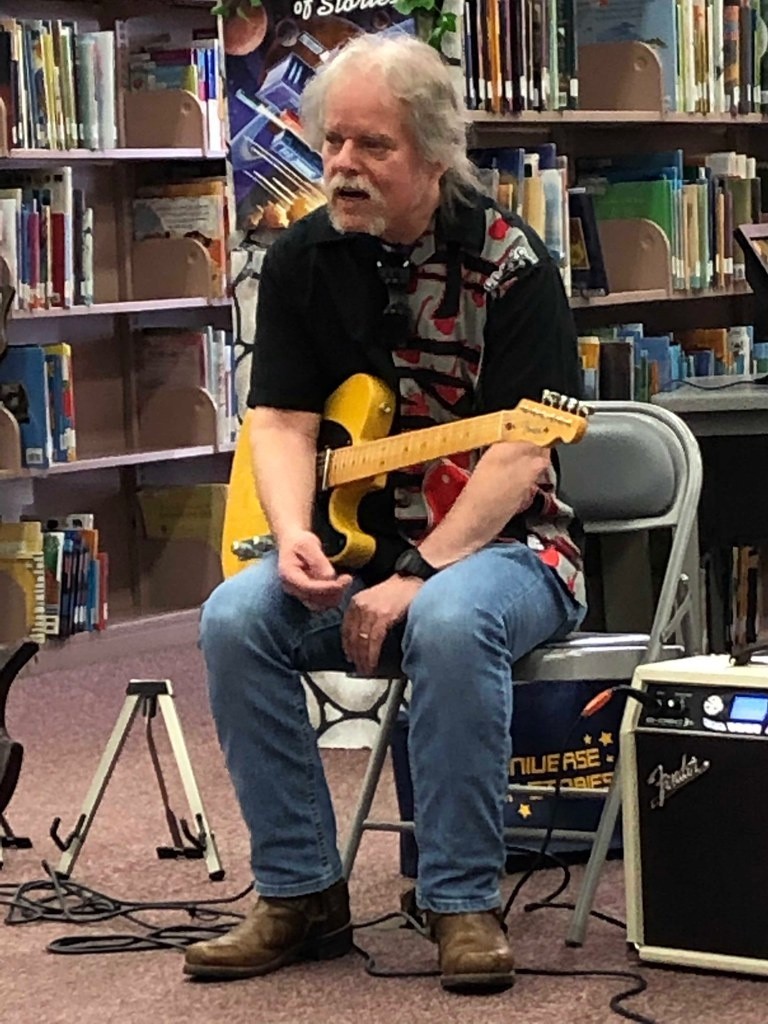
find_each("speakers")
[390,672,623,879]
[620,650,768,978]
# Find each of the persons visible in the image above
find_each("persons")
[182,36,583,993]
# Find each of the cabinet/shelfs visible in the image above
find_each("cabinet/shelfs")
[0,0,768,678]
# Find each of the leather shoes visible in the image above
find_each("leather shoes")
[432,908,516,988]
[182,876,354,976]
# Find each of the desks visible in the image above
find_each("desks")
[650,373,768,655]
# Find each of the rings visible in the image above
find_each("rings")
[359,633,369,640]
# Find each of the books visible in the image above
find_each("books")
[0,0,768,642]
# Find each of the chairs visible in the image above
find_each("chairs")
[343,398,704,949]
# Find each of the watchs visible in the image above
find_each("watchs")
[396,549,438,582]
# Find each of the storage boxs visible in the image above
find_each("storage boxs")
[142,537,224,610]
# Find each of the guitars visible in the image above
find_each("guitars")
[221,372,594,580]
